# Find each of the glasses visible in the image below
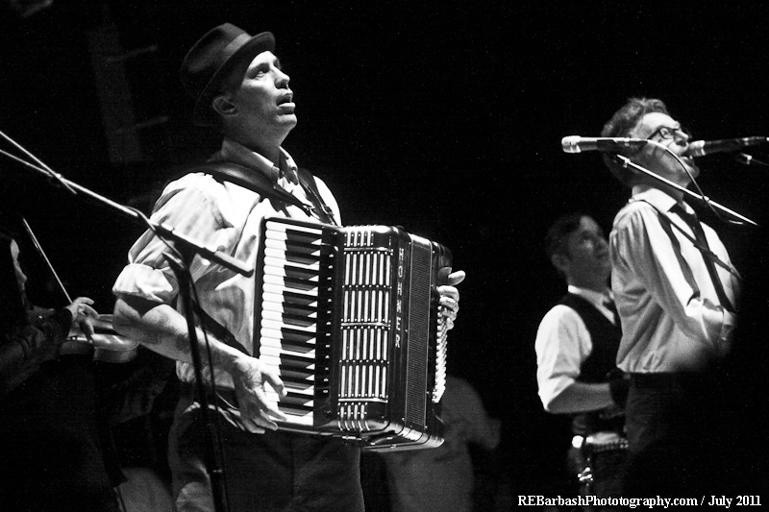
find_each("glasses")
[631,124,682,157]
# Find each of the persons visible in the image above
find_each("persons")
[2,231,176,510]
[534,213,629,508]
[380,375,514,512]
[112,24,468,510]
[593,96,751,511]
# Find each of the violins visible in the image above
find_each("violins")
[27,305,141,363]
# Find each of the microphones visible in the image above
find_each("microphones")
[562,136,668,154]
[688,135,769,158]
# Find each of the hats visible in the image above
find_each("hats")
[177,21,279,122]
